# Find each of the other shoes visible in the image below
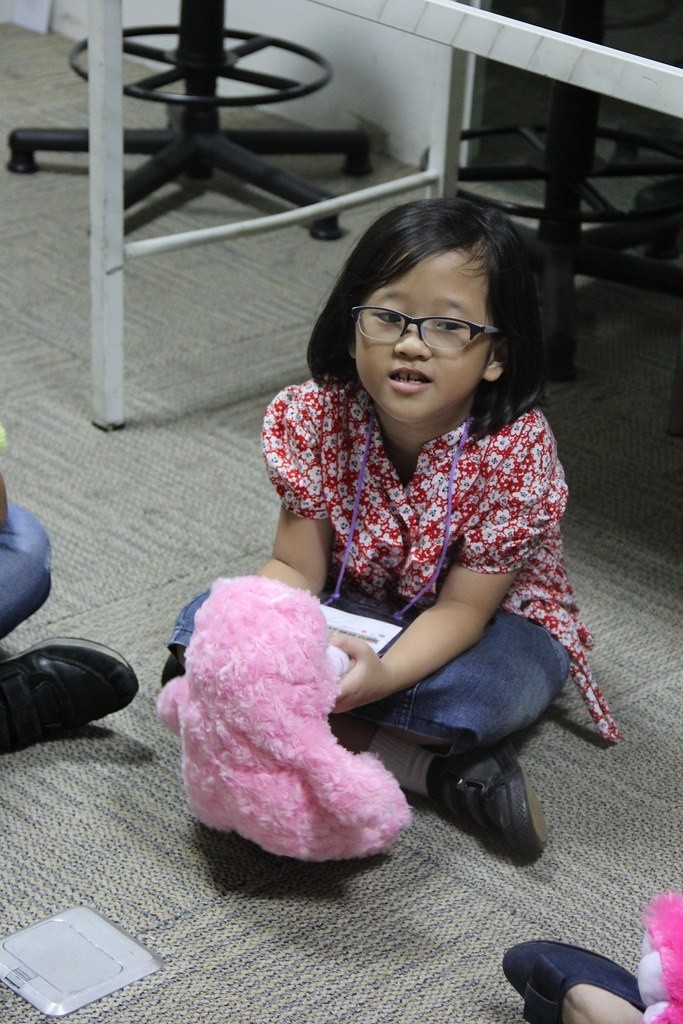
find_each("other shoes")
[500,940,647,1024]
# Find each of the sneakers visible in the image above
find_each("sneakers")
[423,741,546,861]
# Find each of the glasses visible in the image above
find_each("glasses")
[345,305,503,352]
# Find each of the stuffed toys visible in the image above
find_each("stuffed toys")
[155,574,410,864]
[635,891,683,1024]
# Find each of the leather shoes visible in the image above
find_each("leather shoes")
[0,638,140,754]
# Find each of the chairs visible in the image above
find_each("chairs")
[420,2,683,380]
[7,1,375,242]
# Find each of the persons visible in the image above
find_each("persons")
[163,196,625,865]
[501,939,647,1024]
[0,426,139,756]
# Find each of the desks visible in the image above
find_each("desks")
[86,0,683,427]
